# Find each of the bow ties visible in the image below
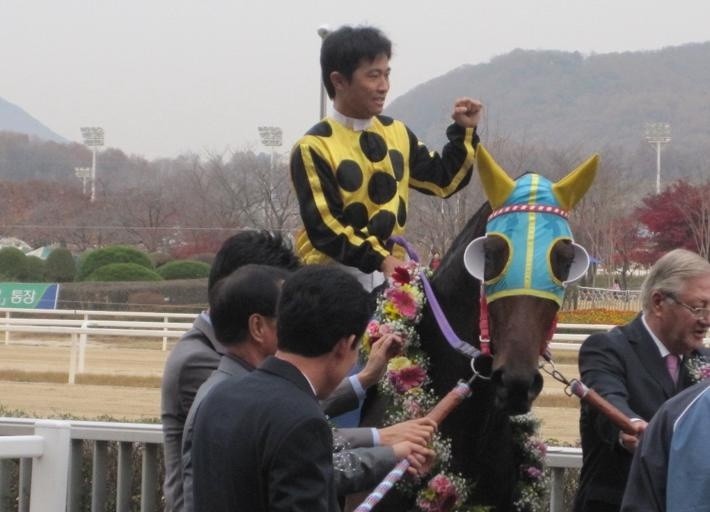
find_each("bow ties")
[351,118,374,132]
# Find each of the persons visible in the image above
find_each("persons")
[191,261,379,512]
[574,247,710,512]
[621,371,710,512]
[289,24,483,310]
[159,224,439,512]
[180,262,439,512]
[613,280,620,299]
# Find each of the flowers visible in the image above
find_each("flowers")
[686,353,709,381]
[360,256,553,512]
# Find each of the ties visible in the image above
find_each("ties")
[661,352,684,395]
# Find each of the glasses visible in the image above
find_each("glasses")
[667,292,710,322]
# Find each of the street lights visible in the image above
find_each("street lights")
[645,122,673,194]
[258,126,284,200]
[74,125,104,201]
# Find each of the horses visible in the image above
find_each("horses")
[339,139,605,512]
